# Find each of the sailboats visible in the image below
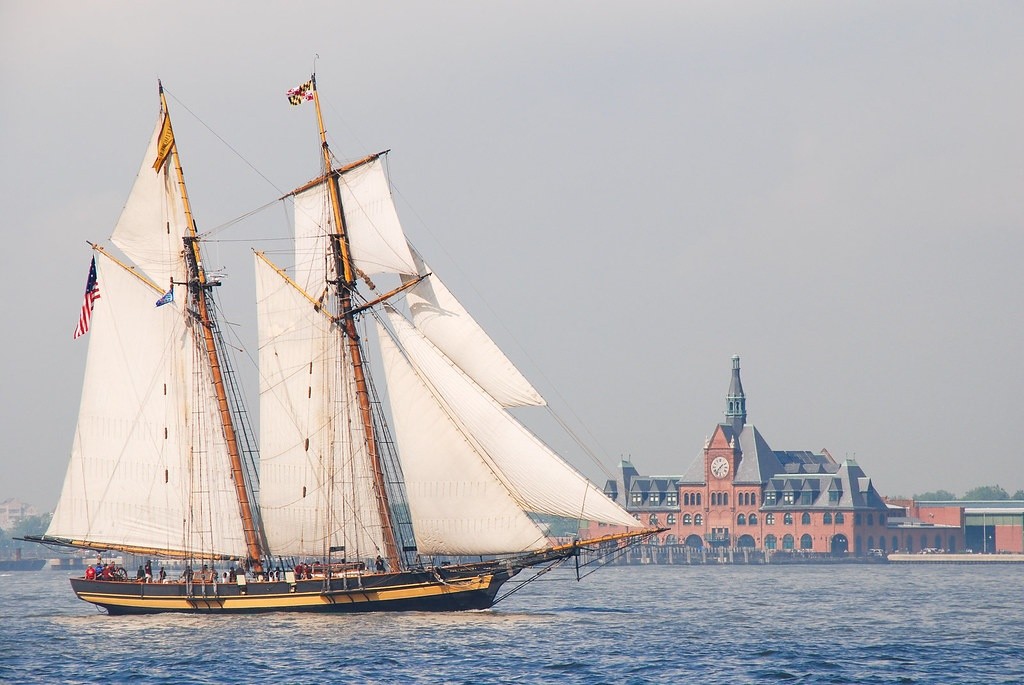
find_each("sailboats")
[11,55,671,613]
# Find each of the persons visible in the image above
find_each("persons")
[375,555,386,574]
[222,571,230,584]
[294,561,311,580]
[160,566,166,582]
[136,559,152,583]
[85,561,121,581]
[183,566,194,583]
[229,567,236,582]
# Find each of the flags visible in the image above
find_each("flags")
[74,253,103,340]
[286,79,314,106]
[151,104,175,173]
[155,284,174,307]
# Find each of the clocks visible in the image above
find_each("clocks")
[711,456,729,479]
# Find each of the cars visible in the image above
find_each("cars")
[868,548,973,556]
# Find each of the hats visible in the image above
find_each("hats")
[145,560,152,563]
